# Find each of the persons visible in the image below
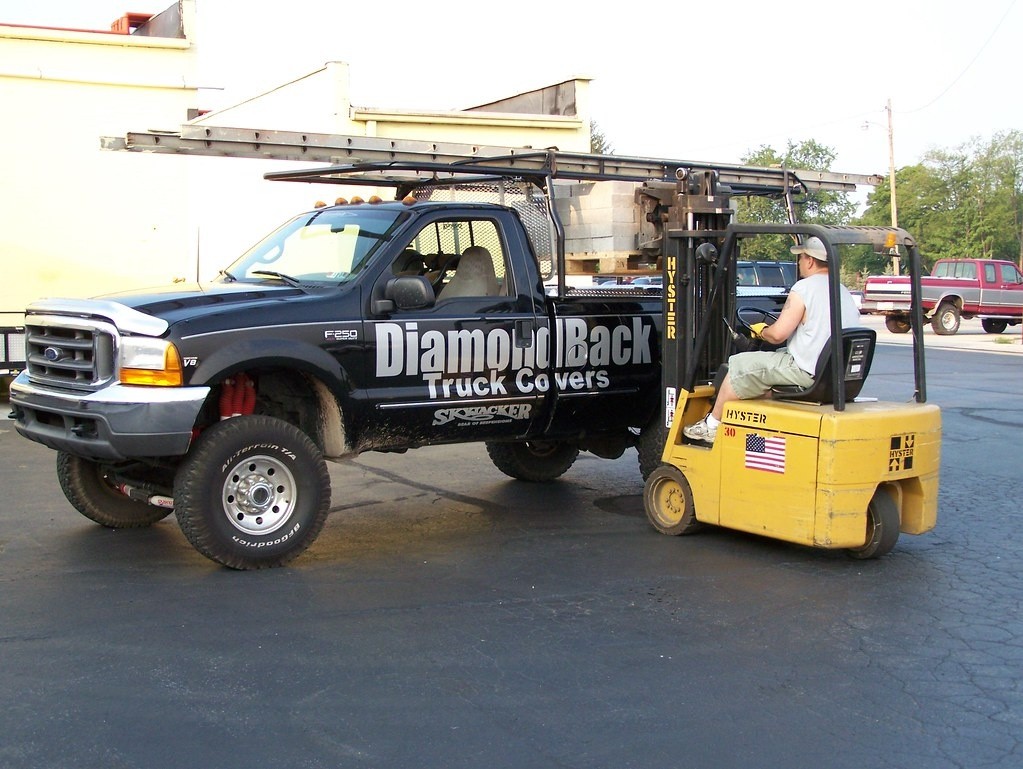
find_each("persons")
[683,236,860,442]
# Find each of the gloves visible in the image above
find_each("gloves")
[750,323,769,341]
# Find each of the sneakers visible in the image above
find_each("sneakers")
[683,413,717,442]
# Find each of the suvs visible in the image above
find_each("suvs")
[602,260,803,337]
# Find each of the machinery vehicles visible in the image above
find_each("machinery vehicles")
[633,169,943,559]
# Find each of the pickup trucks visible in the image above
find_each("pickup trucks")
[7,160,793,572]
[863,259,1023,335]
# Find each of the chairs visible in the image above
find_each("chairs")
[772,327,876,403]
[435,245,499,302]
[392,249,422,276]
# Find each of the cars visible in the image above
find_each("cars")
[850,292,868,314]
[544,274,663,290]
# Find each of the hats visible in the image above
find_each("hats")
[790,236,829,261]
